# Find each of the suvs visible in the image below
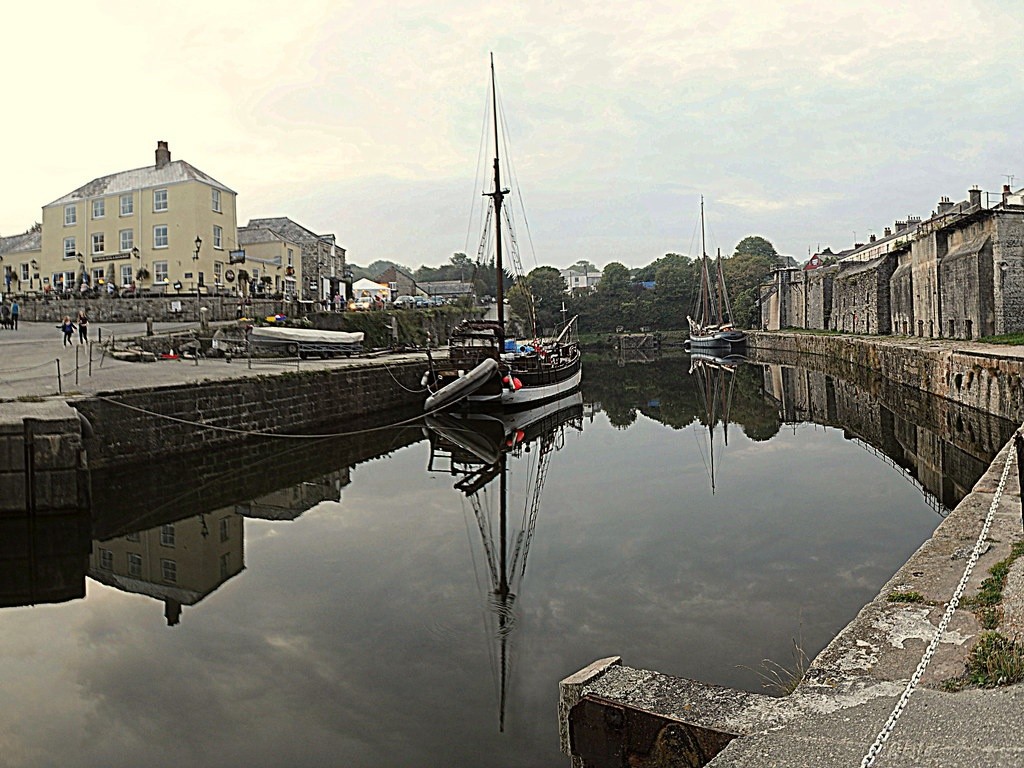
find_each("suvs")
[428,295,447,307]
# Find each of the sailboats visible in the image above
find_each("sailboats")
[422,404,584,733]
[421,39,584,410]
[688,348,747,495]
[686,192,747,348]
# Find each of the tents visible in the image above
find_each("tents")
[352,278,391,304]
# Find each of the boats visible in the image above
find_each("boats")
[425,416,500,464]
[424,358,498,411]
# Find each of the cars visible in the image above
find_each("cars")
[415,298,430,308]
[391,296,416,310]
[350,297,382,312]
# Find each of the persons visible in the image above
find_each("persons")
[0,299,20,330]
[43,277,143,299]
[235,290,388,318]
[55,315,77,348]
[77,310,89,346]
